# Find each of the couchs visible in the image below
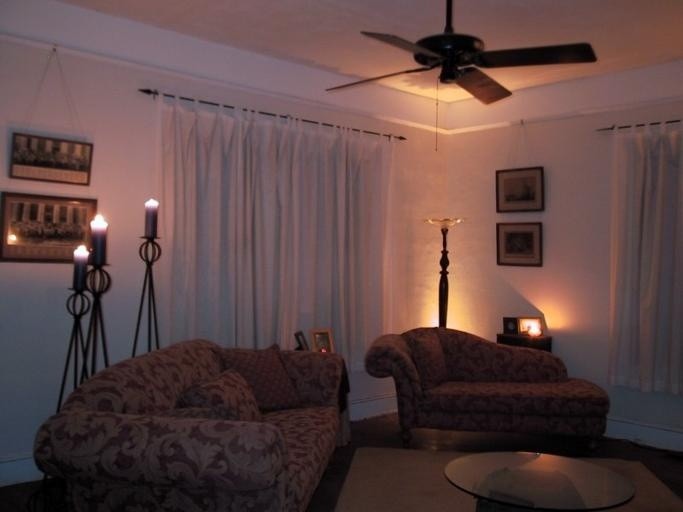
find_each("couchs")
[364,328,609,459]
[32,338,349,511]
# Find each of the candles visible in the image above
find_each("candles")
[73,245,89,289]
[144,197,159,237]
[89,214,108,263]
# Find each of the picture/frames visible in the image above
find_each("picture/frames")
[294,331,309,351]
[0,191,97,263]
[517,317,543,336]
[310,328,334,352]
[495,222,543,267]
[10,132,94,186]
[494,166,544,213]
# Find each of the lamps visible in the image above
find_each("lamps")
[434,55,461,153]
[528,327,542,337]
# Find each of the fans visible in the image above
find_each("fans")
[325,1,596,106]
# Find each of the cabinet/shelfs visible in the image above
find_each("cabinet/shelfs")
[496,334,551,352]
